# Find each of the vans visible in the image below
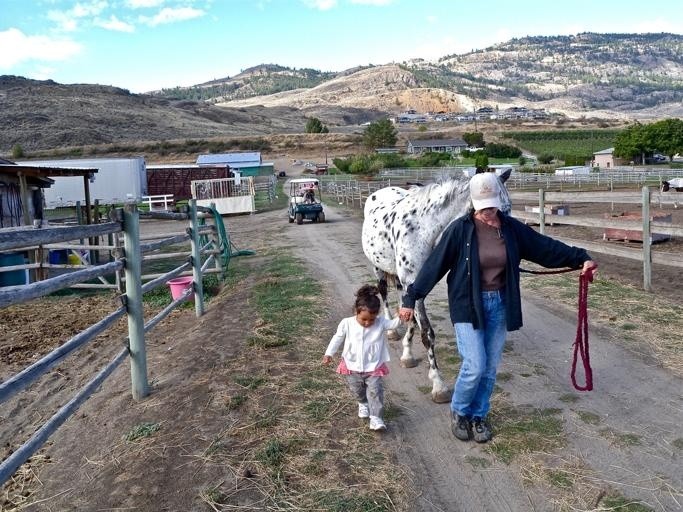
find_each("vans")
[652,154,665,161]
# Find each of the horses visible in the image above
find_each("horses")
[360,167,516,403]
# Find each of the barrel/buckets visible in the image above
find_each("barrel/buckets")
[69,254,80,265]
[167,276,193,302]
[49,248,67,264]
[0,253,26,287]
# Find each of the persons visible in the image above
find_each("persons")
[323,284,410,431]
[399,170,598,443]
[296,181,317,197]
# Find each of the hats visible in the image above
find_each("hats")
[469,172,502,210]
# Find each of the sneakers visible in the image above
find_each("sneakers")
[450,407,471,440]
[358,403,369,418]
[472,416,491,442]
[369,415,387,431]
